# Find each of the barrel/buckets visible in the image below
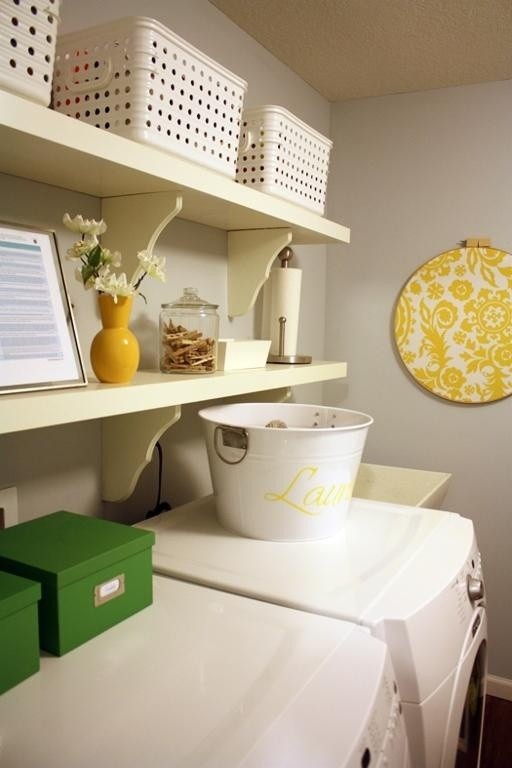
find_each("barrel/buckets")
[200,401,373,543]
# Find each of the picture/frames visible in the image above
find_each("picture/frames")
[1,219,88,401]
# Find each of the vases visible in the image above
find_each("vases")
[89,292,140,384]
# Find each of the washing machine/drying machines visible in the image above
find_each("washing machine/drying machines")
[0,570,416,768]
[135,488,490,768]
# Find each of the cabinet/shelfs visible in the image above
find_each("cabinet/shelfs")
[0,92,351,503]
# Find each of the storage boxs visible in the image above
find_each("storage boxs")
[0,509,155,692]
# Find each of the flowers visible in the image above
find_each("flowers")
[63,210,165,303]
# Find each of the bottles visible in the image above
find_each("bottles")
[158,286,221,376]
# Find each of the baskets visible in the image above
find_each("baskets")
[1,0,333,216]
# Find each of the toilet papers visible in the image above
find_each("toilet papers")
[261,268,302,357]
